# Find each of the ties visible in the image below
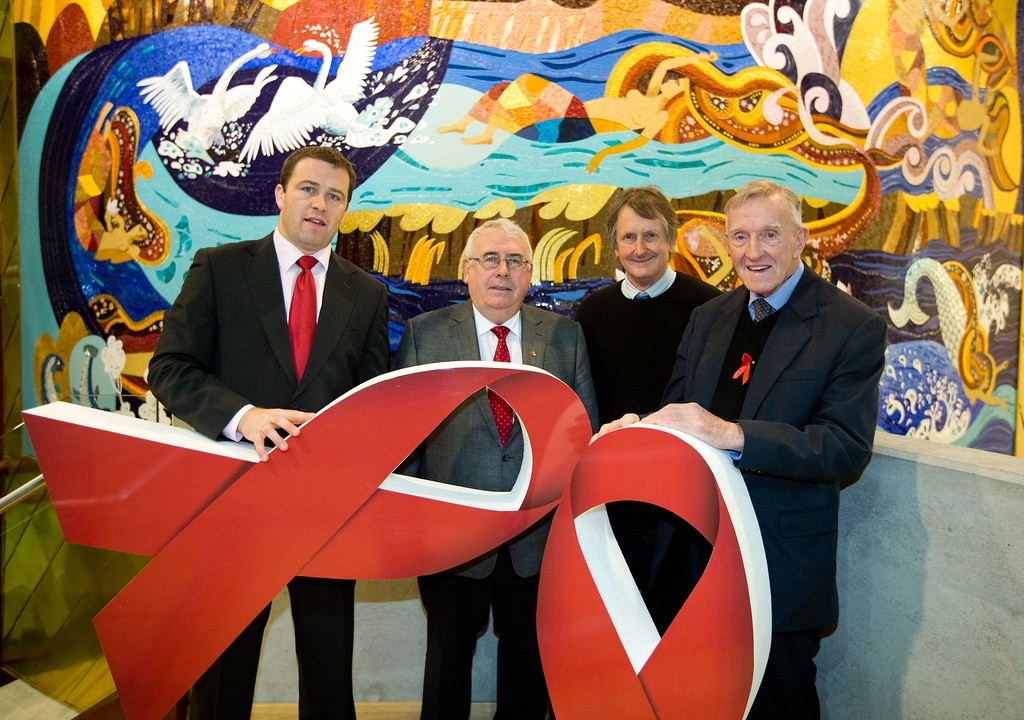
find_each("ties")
[488,326,513,448]
[752,299,772,323]
[288,256,319,386]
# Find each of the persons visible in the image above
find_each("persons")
[147,146,391,720]
[395,218,597,720]
[573,179,888,720]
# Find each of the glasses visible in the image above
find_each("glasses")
[468,254,529,270]
[724,228,794,247]
